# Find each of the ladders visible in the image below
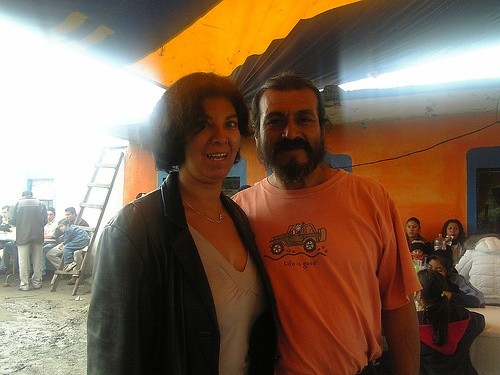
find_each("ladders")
[50,149,123,295]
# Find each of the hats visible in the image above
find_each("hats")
[411,240,425,252]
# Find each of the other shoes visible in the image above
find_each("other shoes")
[42,269,46,276]
[20,281,31,291]
[32,275,43,289]
[63,262,77,272]
[67,277,81,285]
[0,268,8,275]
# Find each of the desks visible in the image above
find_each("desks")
[0,239,56,286]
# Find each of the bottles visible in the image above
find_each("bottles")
[411,255,418,274]
[434,234,446,250]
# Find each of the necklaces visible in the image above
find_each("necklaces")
[182,196,224,224]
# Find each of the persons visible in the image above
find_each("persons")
[427,249,486,308]
[8,190,48,290]
[454,234,500,305]
[46,207,91,279]
[409,239,430,266]
[42,207,59,276]
[57,219,90,271]
[87,72,278,375]
[0,205,17,278]
[229,72,421,375]
[416,268,485,375]
[404,217,433,260]
[432,218,468,264]
